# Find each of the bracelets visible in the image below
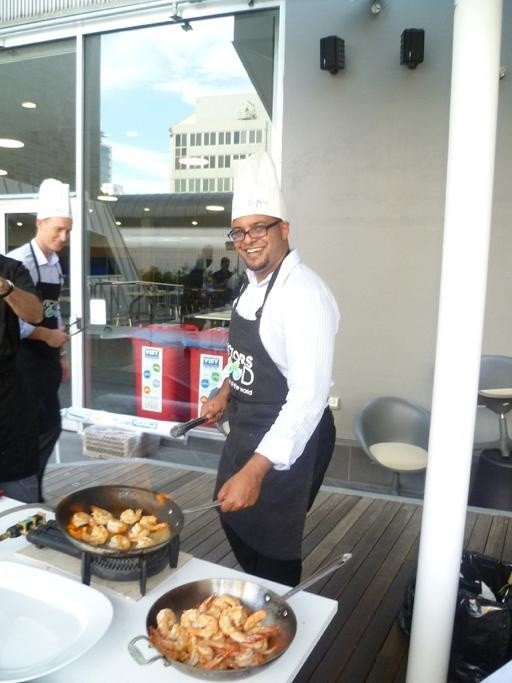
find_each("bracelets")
[0,279,15,299]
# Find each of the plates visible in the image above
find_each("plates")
[0,560,114,681]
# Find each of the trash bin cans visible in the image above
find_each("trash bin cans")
[127,322,199,423]
[399,546,511,682]
[186,326,231,428]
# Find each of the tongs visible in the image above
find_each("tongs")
[62,316,90,339]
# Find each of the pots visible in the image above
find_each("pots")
[55,485,222,557]
[127,551,353,682]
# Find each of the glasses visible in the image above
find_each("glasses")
[227,220,284,244]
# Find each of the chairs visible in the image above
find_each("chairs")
[478,355,512,469]
[352,396,431,496]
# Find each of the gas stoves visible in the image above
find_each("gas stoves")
[79,534,180,602]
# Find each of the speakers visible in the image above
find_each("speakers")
[319,35,345,74]
[400,28,425,70]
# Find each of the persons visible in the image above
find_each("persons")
[202,151,341,587]
[1,251,45,502]
[6,176,73,501]
[213,256,233,288]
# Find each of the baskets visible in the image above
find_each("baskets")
[82,428,161,460]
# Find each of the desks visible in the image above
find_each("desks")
[194,310,232,327]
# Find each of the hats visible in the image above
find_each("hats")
[203,246,213,260]
[196,254,207,270]
[231,159,288,221]
[36,178,73,222]
